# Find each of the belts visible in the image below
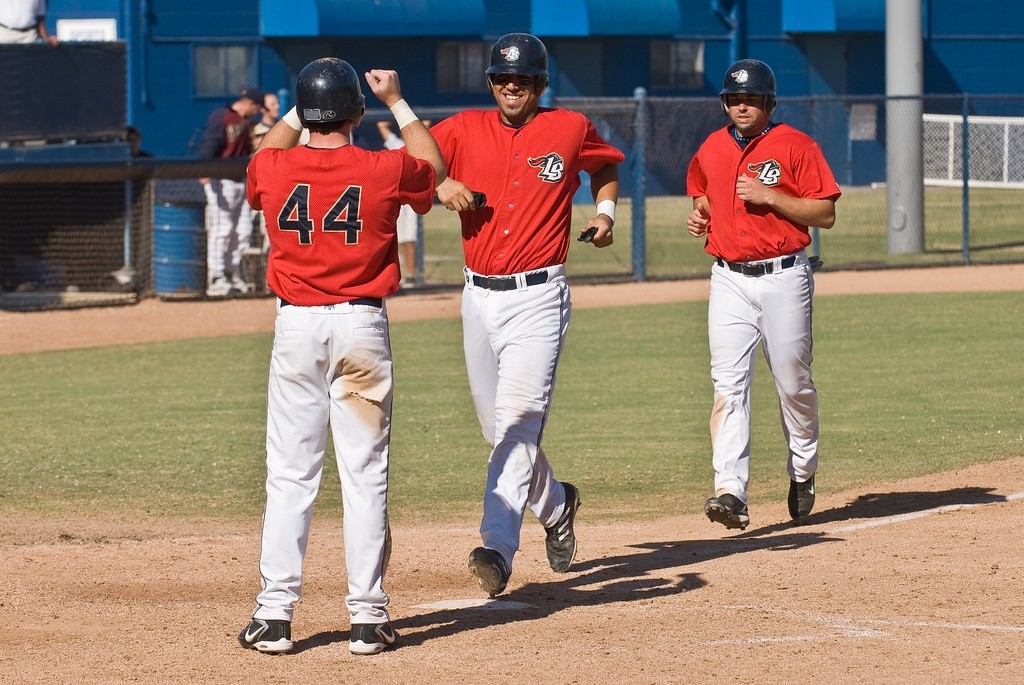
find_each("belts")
[467,270,548,292]
[281,298,384,308]
[717,255,795,277]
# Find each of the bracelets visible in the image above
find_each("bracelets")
[389,98,419,130]
[596,200,616,224]
[282,105,303,132]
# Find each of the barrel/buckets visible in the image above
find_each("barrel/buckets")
[153,203,207,294]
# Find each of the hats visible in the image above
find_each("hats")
[242,89,270,112]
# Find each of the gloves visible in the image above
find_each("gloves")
[808,255,824,272]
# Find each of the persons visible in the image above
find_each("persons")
[0,0,61,48]
[687,60,843,531]
[376,120,443,287]
[197,88,269,298]
[397,33,625,599]
[238,57,447,657]
[236,94,280,255]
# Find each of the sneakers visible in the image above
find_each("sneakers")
[788,472,815,519]
[467,547,511,596]
[544,482,582,572]
[704,494,750,530]
[237,619,294,652]
[348,624,400,655]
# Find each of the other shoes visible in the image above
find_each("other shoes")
[232,276,254,297]
[207,278,233,297]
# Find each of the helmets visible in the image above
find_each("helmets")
[296,57,366,127]
[718,59,776,116]
[484,32,549,97]
